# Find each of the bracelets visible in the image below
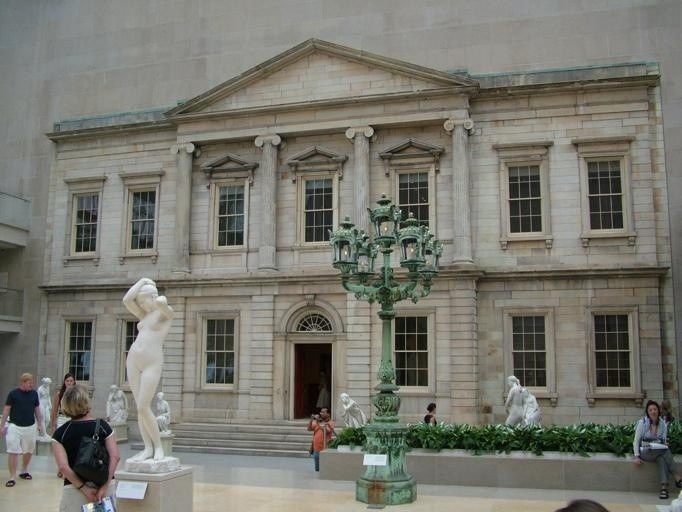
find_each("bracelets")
[74,480,88,490]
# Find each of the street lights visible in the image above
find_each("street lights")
[329,193,445,505]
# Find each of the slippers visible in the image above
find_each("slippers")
[20,473,32,479]
[6,480,15,486]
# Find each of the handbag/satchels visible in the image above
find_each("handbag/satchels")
[74,417,110,488]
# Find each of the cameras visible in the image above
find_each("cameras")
[312,415,316,418]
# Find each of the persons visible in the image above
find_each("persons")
[306,406,334,473]
[0,373,44,486]
[632,400,681,498]
[81,349,89,378]
[423,403,439,424]
[38,377,54,438]
[500,375,524,426]
[104,384,129,422]
[313,370,330,408]
[521,389,540,428]
[155,391,172,432]
[52,373,79,478]
[50,384,119,511]
[120,277,174,459]
[550,499,611,512]
[338,392,367,428]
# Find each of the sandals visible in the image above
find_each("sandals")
[659,488,668,498]
[675,478,682,487]
